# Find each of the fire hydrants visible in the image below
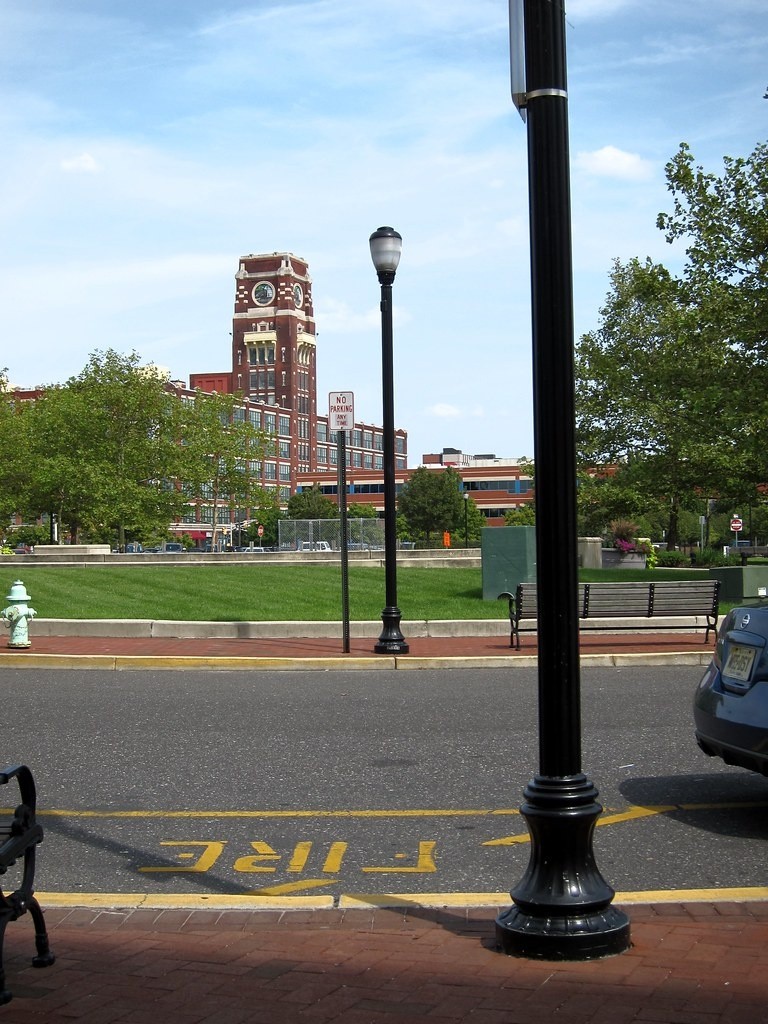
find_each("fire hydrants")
[1,580,37,648]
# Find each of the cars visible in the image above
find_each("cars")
[693,599,768,778]
[650,538,754,549]
[113,538,415,554]
[10,543,35,555]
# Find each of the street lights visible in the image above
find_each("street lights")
[369,226,410,654]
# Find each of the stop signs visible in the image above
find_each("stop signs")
[258,525,264,537]
[730,519,742,531]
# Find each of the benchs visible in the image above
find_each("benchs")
[498,578,722,651]
